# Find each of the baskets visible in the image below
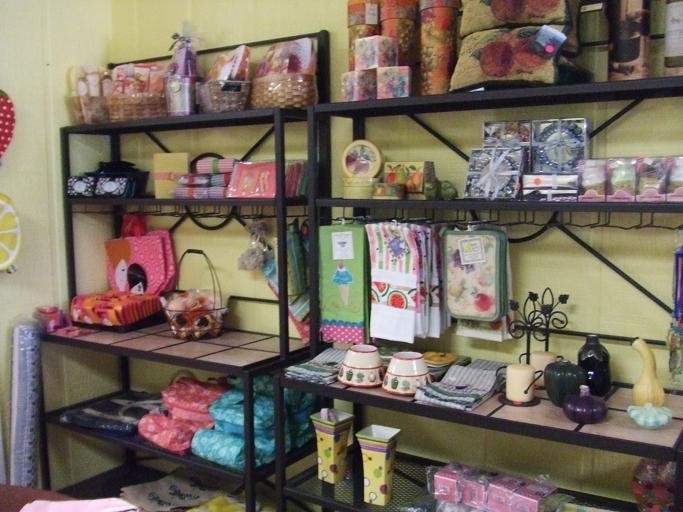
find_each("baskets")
[77,71,110,124]
[194,79,250,114]
[158,249,231,339]
[250,71,316,111]
[107,92,168,121]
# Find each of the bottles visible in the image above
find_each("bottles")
[542,334,618,424]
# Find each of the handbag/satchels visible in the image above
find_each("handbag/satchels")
[66,160,149,198]
[256,216,308,346]
[60,391,167,437]
[68,214,177,328]
[137,371,319,469]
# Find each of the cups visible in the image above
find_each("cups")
[335,345,385,389]
[382,351,432,396]
